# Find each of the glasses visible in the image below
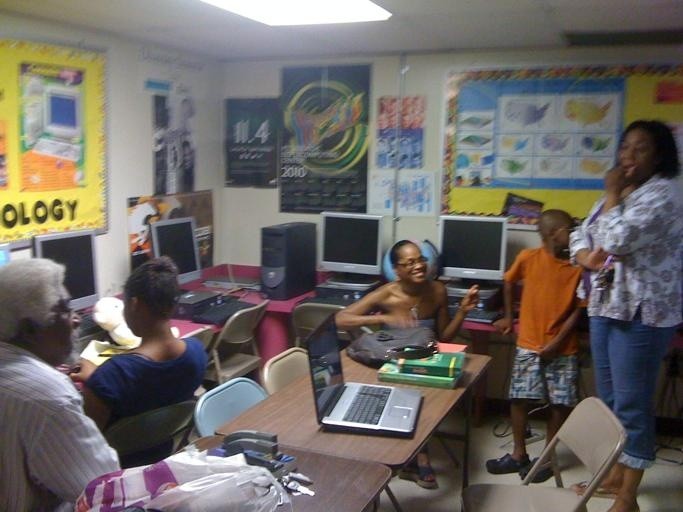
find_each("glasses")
[397,257,429,269]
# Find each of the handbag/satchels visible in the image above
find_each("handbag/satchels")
[574,265,592,301]
[344,326,439,371]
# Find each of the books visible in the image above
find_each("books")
[377,358,464,389]
[398,347,466,377]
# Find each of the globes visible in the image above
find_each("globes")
[384,239,443,283]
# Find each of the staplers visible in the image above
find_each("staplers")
[220,430,283,472]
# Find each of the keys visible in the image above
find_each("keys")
[287,480,315,496]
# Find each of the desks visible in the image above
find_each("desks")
[215,348,494,510]
[168,434,402,512]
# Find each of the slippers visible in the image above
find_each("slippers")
[485,453,532,475]
[398,464,436,489]
[520,457,555,484]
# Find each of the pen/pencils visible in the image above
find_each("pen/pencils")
[99,354,115,357]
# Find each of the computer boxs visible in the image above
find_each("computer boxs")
[171,289,223,320]
[260,221,316,300]
[314,284,383,300]
[445,287,504,313]
[23,78,43,145]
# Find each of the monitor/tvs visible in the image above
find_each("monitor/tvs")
[314,211,384,287]
[43,85,80,141]
[150,216,202,298]
[0,243,10,267]
[435,215,507,293]
[34,230,99,319]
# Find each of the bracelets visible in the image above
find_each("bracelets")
[458,306,468,313]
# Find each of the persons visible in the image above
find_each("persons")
[487,210,589,483]
[0,258,120,512]
[69,257,208,468]
[568,120,683,512]
[334,240,480,488]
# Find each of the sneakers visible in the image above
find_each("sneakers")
[568,481,620,500]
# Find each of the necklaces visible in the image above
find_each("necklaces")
[408,304,421,320]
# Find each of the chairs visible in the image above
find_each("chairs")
[104,399,198,470]
[349,325,372,343]
[209,299,271,386]
[460,396,630,510]
[261,347,310,394]
[193,377,269,437]
[292,303,346,349]
[181,324,213,351]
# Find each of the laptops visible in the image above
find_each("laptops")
[305,313,423,437]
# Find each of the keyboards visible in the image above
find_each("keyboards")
[33,140,81,162]
[316,295,353,307]
[192,299,257,328]
[448,307,500,323]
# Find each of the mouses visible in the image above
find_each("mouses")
[76,170,83,182]
[64,367,79,379]
